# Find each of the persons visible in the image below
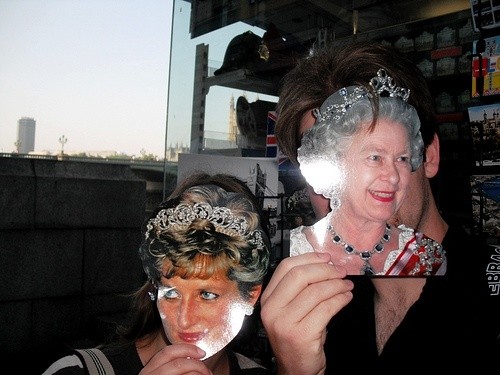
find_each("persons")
[260,41,500,375]
[140,184,268,362]
[42,175,274,375]
[290,69,446,275]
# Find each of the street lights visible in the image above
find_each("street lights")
[59,135,67,156]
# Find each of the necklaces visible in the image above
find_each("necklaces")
[329,223,391,275]
